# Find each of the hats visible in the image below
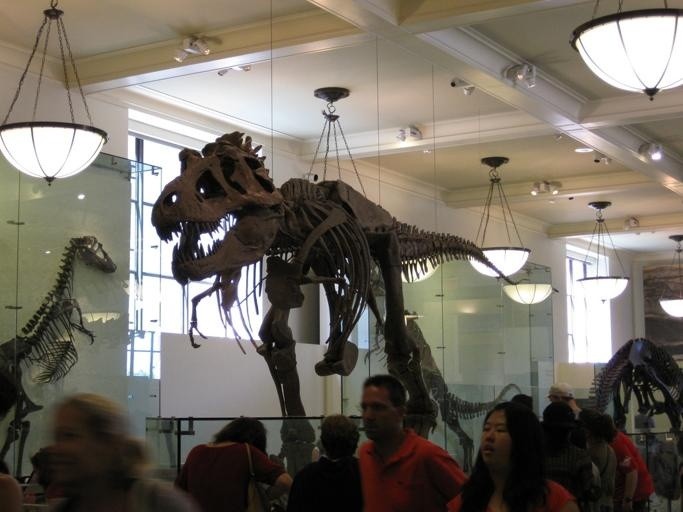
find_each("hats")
[543,382,572,399]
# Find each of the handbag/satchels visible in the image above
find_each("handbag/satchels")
[247,444,265,512]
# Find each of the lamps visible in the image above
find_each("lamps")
[0,0,109,187]
[563,2,683,101]
[467,155,681,321]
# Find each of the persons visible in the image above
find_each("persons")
[48,394,199,511]
[356,375,469,512]
[446,403,579,511]
[288,416,362,512]
[513,383,654,512]
[29,444,68,512]
[0,473,21,512]
[174,417,293,511]
